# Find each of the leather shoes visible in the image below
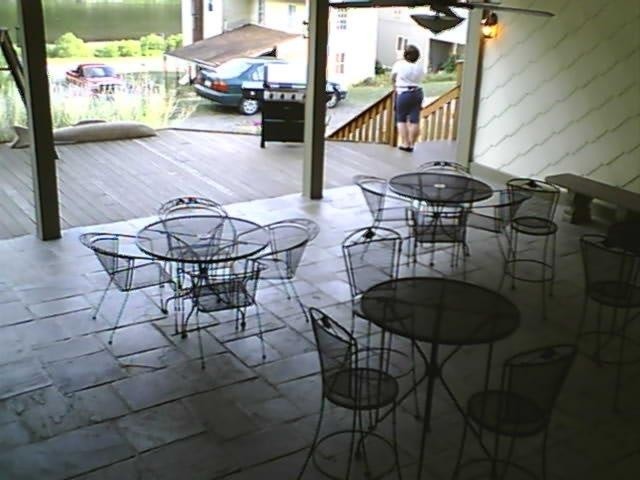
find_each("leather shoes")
[399,147,410,153]
[411,147,414,151]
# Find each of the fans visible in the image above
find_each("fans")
[328,0,555,36]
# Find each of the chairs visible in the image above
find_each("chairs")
[506,176,561,299]
[416,159,473,255]
[157,194,238,288]
[79,231,177,345]
[340,225,404,363]
[450,342,584,480]
[353,174,429,269]
[455,188,532,270]
[236,217,320,324]
[573,234,639,376]
[176,257,268,373]
[295,304,403,479]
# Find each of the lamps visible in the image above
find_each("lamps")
[479,14,499,38]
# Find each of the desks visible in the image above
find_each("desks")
[389,172,495,265]
[134,215,270,331]
[353,275,538,473]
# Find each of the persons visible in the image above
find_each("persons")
[391,45,424,152]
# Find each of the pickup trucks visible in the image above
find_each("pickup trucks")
[66,64,122,94]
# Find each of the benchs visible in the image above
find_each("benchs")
[544,172,640,227]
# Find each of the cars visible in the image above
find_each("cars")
[194,58,348,115]
